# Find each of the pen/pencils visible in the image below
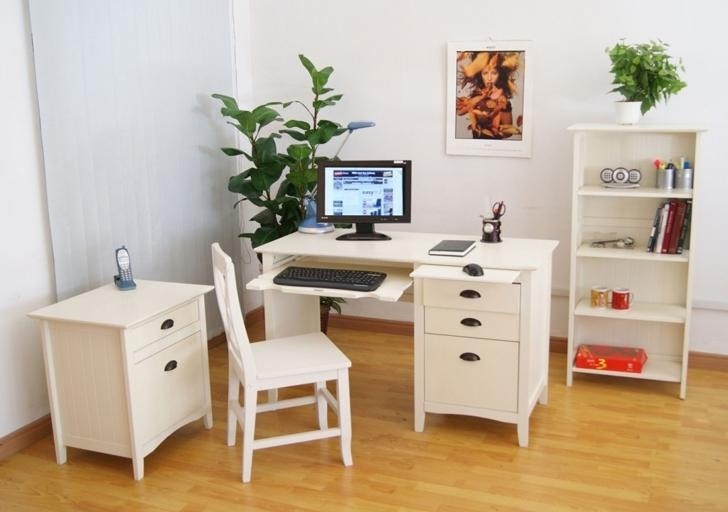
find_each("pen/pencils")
[497,201,503,217]
[655,157,689,169]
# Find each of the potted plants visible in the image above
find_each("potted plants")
[604,34,688,124]
[210,53,349,335]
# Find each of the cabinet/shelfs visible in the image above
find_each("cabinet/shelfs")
[26,279,215,481]
[568,120,709,400]
[244,228,560,447]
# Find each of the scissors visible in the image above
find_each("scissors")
[492,202,505,219]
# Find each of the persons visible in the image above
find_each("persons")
[456,52,512,139]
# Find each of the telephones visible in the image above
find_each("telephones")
[114,246,136,291]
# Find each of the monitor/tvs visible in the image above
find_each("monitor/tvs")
[316,159,411,240]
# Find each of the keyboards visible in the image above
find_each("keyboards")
[273,266,386,292]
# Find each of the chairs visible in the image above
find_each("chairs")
[211,241,354,484]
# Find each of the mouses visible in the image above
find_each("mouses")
[464,264,484,277]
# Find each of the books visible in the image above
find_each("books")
[428,240,476,257]
[646,200,691,254]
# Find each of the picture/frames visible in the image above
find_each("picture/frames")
[444,40,533,159]
